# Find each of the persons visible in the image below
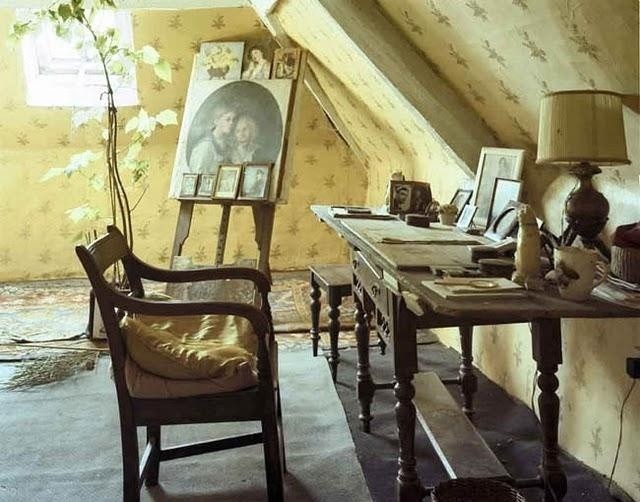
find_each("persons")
[227,116,266,164]
[242,46,270,79]
[393,186,410,211]
[422,278,523,300]
[248,169,264,197]
[189,104,238,174]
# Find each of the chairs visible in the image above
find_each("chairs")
[76,224,286,502]
[309,172,419,383]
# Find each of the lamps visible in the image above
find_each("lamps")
[535,90,632,262]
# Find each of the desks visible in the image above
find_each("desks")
[308,205,640,502]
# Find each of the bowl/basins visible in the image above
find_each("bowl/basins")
[478,258,514,279]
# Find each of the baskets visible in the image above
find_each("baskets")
[432,477,524,501]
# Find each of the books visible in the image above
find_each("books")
[366,229,478,244]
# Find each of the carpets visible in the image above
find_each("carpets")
[1,277,440,350]
[0,347,370,501]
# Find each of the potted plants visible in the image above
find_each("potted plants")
[437,204,458,225]
[6,0,178,342]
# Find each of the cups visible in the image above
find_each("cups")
[553,246,608,302]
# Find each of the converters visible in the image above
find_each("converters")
[626,357,639,377]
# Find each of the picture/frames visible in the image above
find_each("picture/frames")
[469,147,525,230]
[391,180,432,216]
[196,174,218,198]
[238,161,272,200]
[198,40,246,78]
[180,173,198,198]
[451,190,474,222]
[457,203,478,232]
[273,46,300,78]
[486,177,523,232]
[483,200,525,242]
[213,164,243,198]
[242,38,277,79]
